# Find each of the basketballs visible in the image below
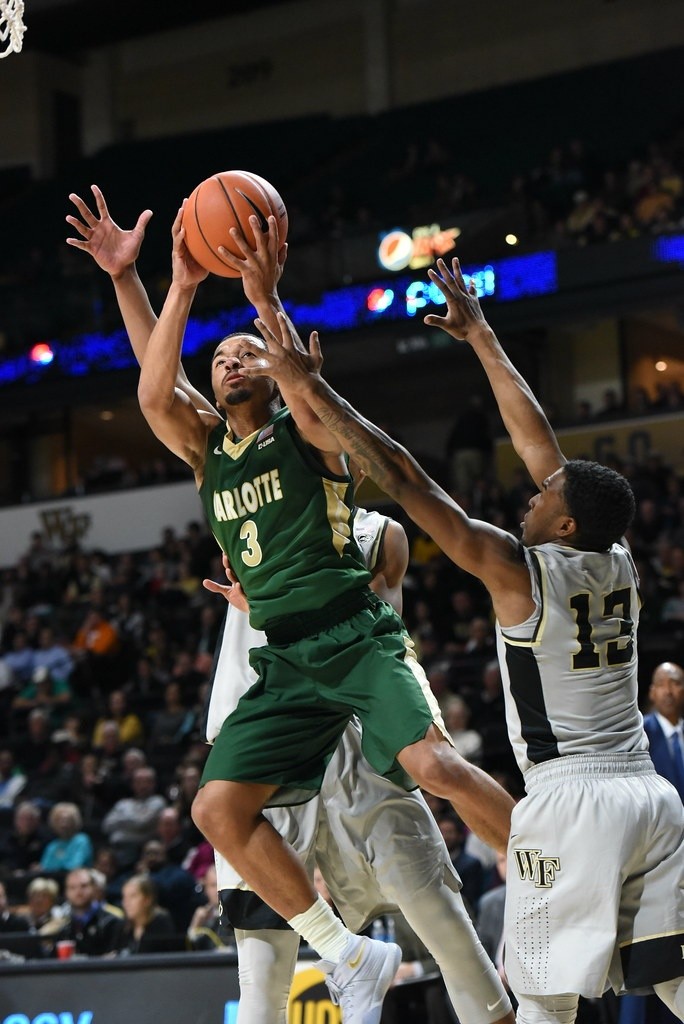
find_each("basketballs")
[182,169,288,279]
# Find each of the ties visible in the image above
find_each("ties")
[670,732,684,778]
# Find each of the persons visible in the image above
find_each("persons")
[0,145,684,1024]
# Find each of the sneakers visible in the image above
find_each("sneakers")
[309,931,403,1024]
